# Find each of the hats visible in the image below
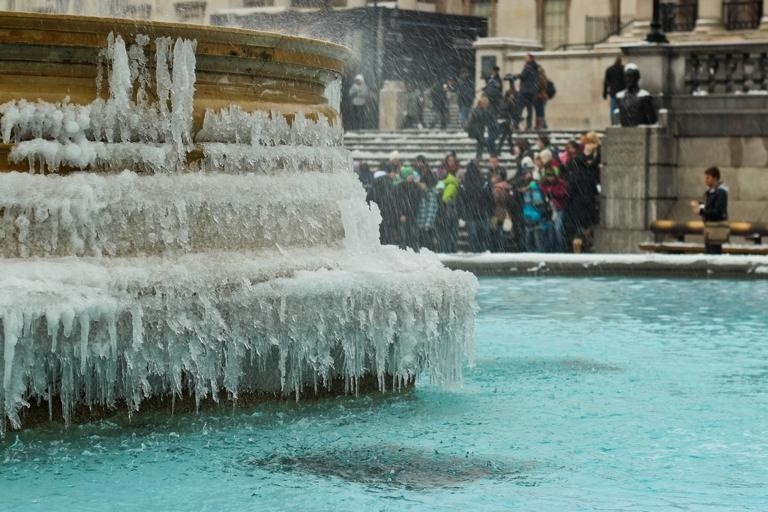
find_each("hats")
[399,166,413,179]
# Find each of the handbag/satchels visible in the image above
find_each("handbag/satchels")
[703,221,731,245]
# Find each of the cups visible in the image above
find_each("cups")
[572,239,582,253]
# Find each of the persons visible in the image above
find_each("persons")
[615,66,659,127]
[689,165,731,255]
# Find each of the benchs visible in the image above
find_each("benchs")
[636,218,768,255]
